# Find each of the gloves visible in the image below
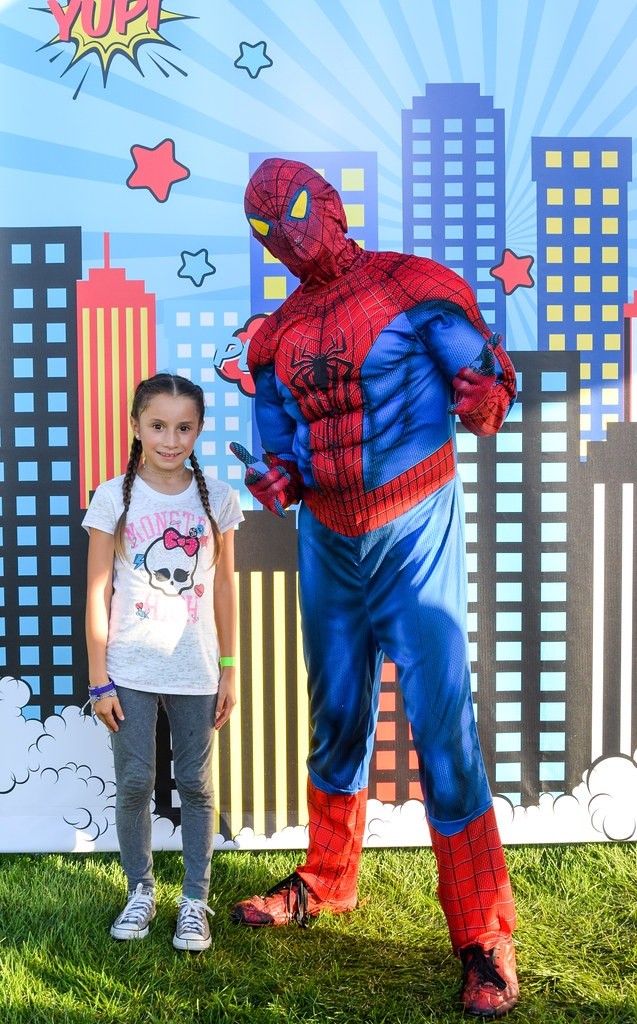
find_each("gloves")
[229,442,292,519]
[447,333,503,416]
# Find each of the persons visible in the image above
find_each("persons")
[81,373,246,953]
[228,157,520,1018]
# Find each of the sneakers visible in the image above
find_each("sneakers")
[110,883,156,939]
[171,893,216,950]
[460,932,520,1017]
[232,869,356,929]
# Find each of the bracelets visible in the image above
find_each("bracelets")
[88,678,116,701]
[219,657,236,668]
